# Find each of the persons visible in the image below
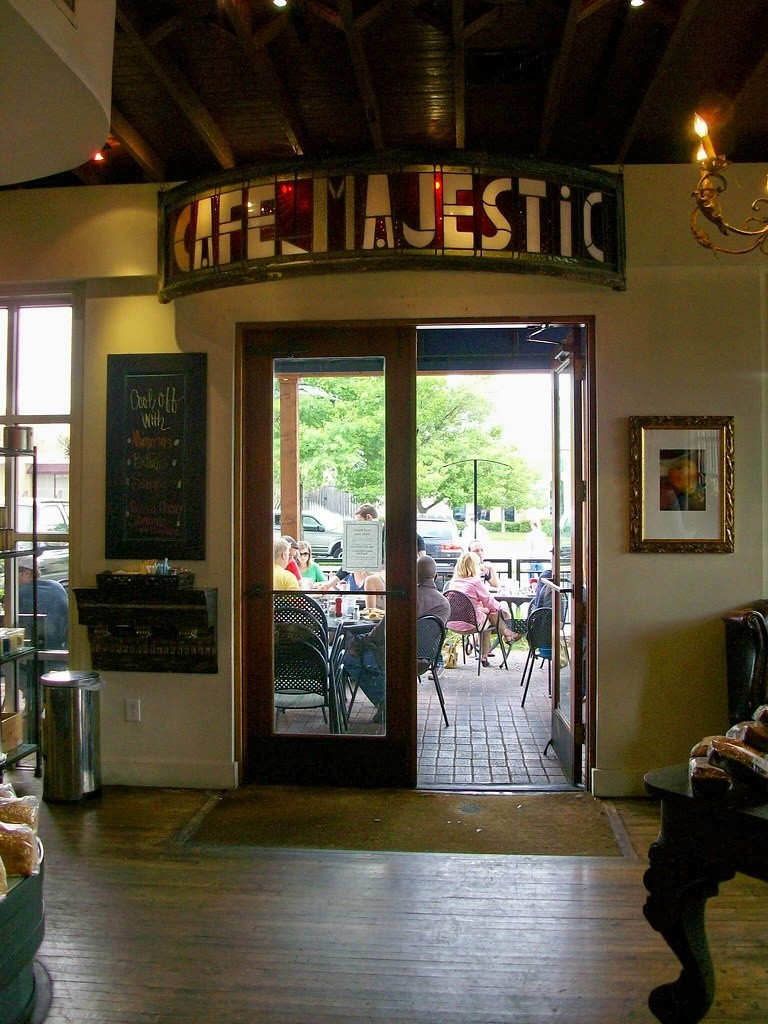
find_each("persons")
[273,504,427,680]
[341,555,451,723]
[449,539,522,667]
[18,556,69,650]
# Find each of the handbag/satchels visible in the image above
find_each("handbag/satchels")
[441,642,458,668]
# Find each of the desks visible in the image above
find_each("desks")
[644,762,768,1024]
[491,589,536,668]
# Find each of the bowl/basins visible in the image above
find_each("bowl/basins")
[348,605,359,613]
[4,423,33,451]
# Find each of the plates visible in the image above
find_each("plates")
[332,619,357,624]
[360,610,386,620]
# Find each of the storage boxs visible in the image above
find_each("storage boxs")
[0,712,24,752]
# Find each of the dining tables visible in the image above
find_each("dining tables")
[323,608,383,633]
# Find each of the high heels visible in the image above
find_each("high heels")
[481,656,490,667]
[505,632,520,646]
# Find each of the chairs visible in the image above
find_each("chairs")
[273,592,349,735]
[443,590,509,676]
[347,614,449,728]
[521,592,569,707]
[722,598,768,727]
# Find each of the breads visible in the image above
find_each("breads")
[687,705,768,789]
[0,786,40,897]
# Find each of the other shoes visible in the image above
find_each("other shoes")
[372,699,385,722]
[428,665,445,679]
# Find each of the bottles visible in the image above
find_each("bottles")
[529,578,537,594]
[353,608,360,620]
[336,598,342,616]
[328,608,334,616]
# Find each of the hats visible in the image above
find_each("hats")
[18,556,37,570]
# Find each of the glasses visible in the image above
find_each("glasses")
[300,552,310,557]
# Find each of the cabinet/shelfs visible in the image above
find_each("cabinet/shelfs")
[0,446,42,784]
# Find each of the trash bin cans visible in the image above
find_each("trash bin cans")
[36,668,103,808]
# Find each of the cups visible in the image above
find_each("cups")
[0,628,24,657]
[364,608,372,612]
[338,581,350,591]
[331,605,335,611]
[500,580,519,596]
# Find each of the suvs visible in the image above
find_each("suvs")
[417,514,464,578]
[273,510,345,559]
[0,496,69,601]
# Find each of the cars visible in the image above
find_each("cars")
[530,545,572,580]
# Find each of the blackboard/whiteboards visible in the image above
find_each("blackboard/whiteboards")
[105,354,208,562]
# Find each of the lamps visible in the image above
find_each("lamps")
[691,112,768,255]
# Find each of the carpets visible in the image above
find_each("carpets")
[183,787,624,858]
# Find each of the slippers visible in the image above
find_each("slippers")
[487,652,495,657]
[466,635,473,656]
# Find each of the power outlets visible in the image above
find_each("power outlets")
[126,699,140,721]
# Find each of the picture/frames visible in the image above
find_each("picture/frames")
[628,415,734,555]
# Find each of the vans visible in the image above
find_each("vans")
[453,508,465,520]
